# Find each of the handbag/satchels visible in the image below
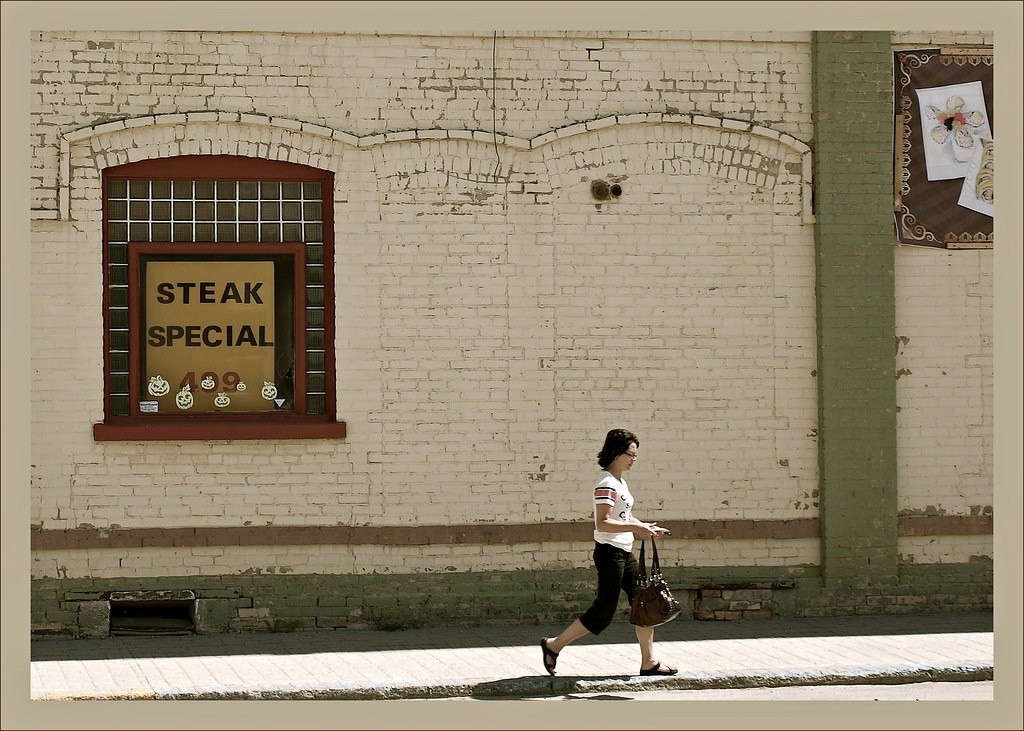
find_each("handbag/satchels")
[629,573,681,628]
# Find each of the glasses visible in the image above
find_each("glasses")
[623,452,637,460]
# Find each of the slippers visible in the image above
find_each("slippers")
[640,660,678,676]
[540,637,559,677]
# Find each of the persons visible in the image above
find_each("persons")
[540,429,678,677]
